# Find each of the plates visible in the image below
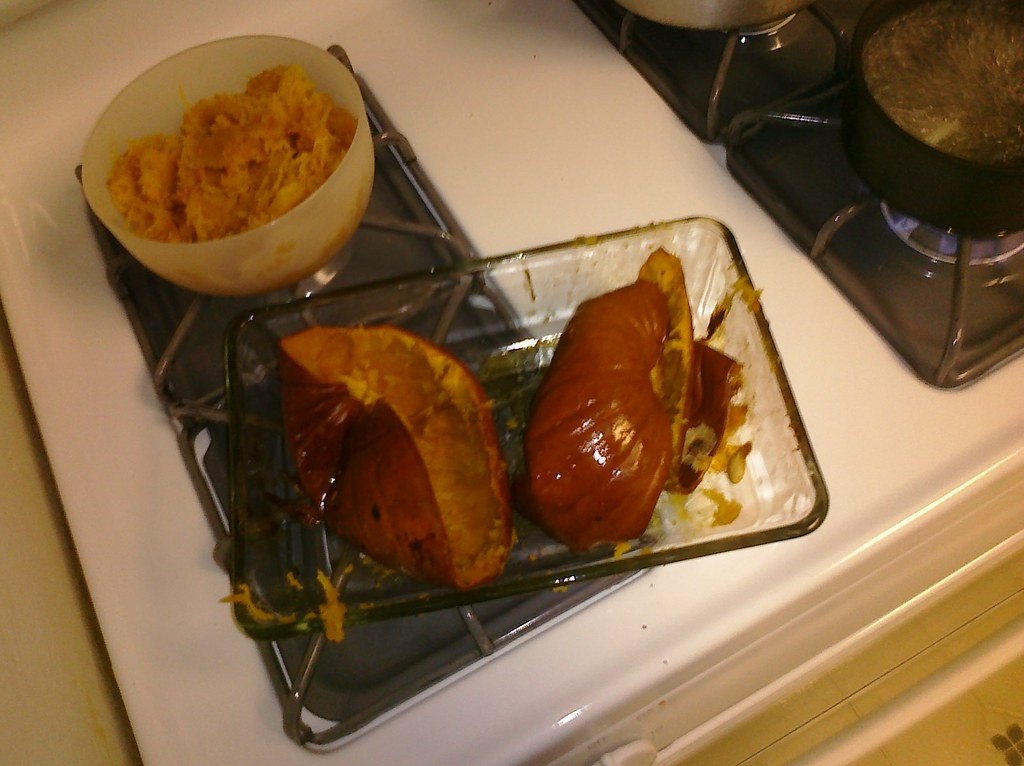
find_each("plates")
[223,214,828,640]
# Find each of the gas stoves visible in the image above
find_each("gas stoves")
[1,0,1024,764]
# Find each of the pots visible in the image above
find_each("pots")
[841,1,1024,240]
[623,1,807,30]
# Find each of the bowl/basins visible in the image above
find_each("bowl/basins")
[79,35,377,300]
